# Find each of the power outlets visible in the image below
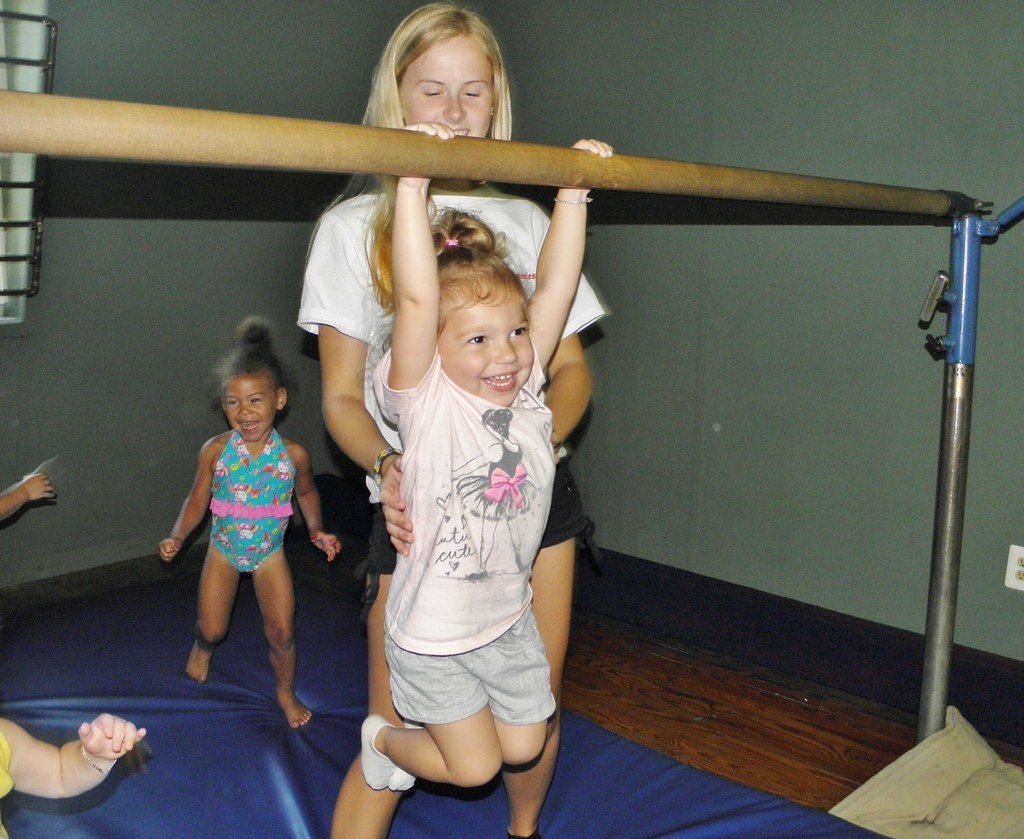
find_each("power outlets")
[1005,545,1024,591]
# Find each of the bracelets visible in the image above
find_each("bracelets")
[373,447,404,486]
[81,743,117,774]
[170,535,182,542]
[554,196,593,204]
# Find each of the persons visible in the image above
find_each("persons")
[360,122,612,792]
[0,713,146,839]
[297,2,605,839]
[158,317,342,727]
[0,474,54,521]
